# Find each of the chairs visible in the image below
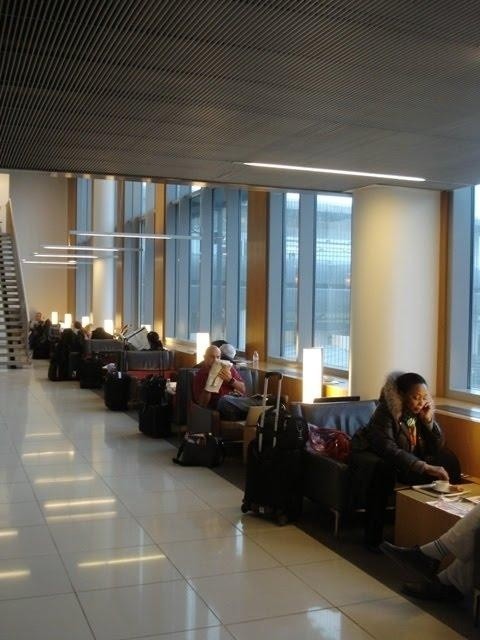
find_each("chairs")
[48,324,396,538]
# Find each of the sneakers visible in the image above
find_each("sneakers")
[380,540,441,577]
[401,576,463,609]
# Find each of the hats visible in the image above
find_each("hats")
[221,343,236,359]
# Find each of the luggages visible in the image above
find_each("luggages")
[104,339,131,412]
[28,326,104,390]
[240,371,298,526]
[138,405,172,438]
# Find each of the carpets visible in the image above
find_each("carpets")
[91,388,480,640]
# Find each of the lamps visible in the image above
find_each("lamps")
[22,231,172,265]
[82,317,89,327]
[51,312,58,325]
[104,320,114,335]
[65,314,71,329]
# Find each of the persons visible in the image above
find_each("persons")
[345,371,450,555]
[140,331,164,351]
[194,344,236,368]
[29,312,114,358]
[193,345,273,421]
[381,502,480,602]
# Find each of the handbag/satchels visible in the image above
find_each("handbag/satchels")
[181,432,226,468]
[255,403,352,462]
[138,374,165,404]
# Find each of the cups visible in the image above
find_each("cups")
[432,480,449,494]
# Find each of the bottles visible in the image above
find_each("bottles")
[253,351,258,368]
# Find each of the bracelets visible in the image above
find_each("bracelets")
[228,378,235,385]
